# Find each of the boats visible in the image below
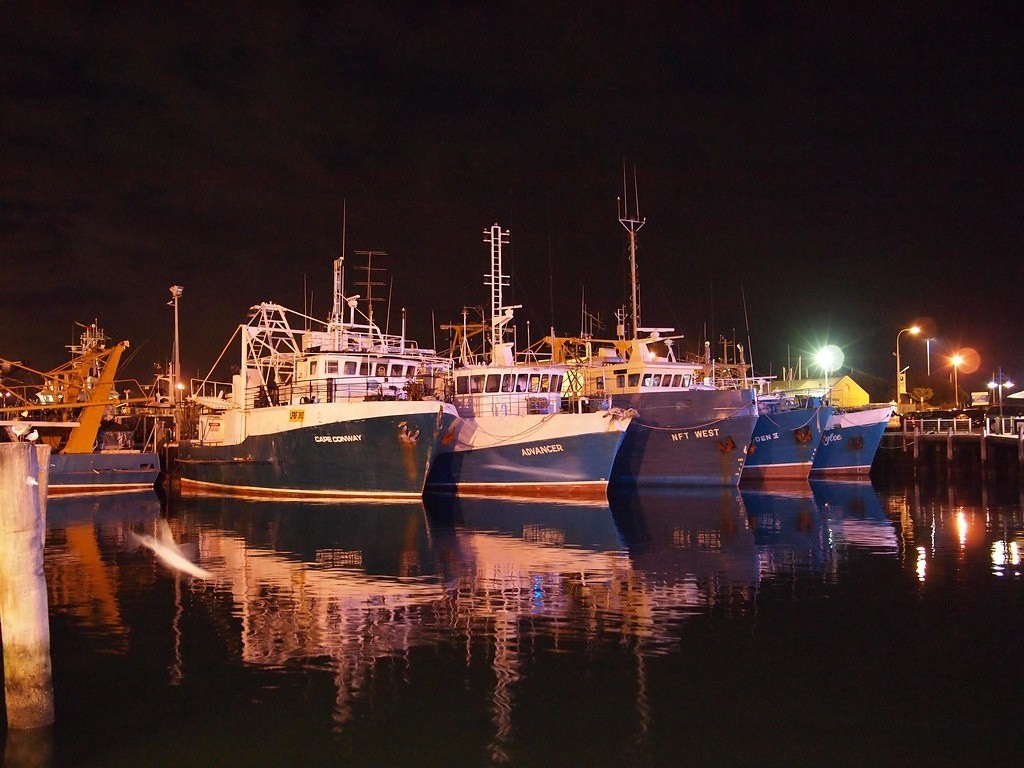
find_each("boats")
[1,156,893,499]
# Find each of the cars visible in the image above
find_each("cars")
[885,404,1024,433]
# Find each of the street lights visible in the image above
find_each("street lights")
[989,366,1013,417]
[897,326,920,403]
[955,356,962,406]
